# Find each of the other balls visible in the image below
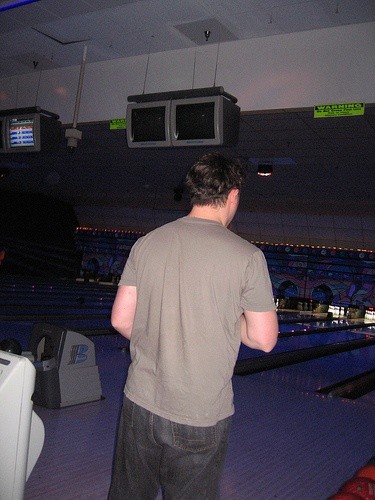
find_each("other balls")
[0,339,22,356]
[326,467,375,500]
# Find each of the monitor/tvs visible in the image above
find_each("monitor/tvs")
[171,96,240,147]
[2,113,62,153]
[126,99,171,148]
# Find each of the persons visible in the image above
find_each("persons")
[108,150,278,500]
